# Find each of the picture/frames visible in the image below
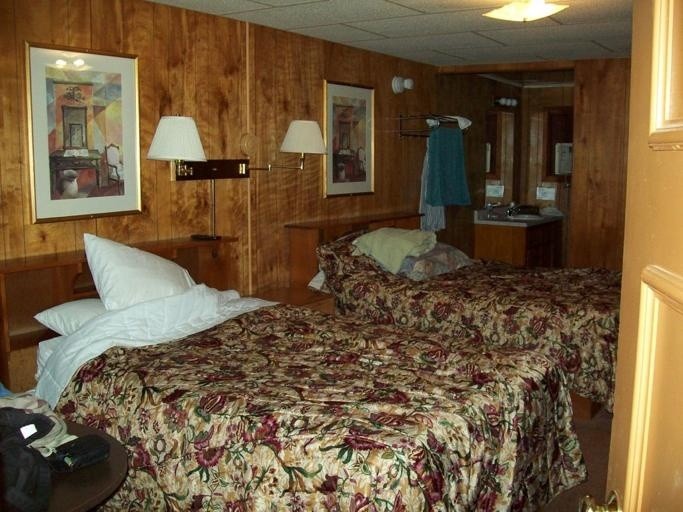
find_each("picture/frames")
[320,79,377,199]
[24,41,143,223]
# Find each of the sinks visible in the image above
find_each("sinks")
[509,214,540,219]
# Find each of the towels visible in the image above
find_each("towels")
[425,126,472,207]
[418,138,447,234]
[426,114,472,130]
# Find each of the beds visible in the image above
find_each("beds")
[286,210,618,424]
[1,235,590,512]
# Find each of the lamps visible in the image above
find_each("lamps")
[494,98,520,107]
[238,119,327,174]
[392,75,414,95]
[148,115,208,183]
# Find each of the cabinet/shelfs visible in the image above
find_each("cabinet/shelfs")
[472,221,563,267]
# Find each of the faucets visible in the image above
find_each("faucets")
[486,201,502,218]
[509,198,521,216]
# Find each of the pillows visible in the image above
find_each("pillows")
[398,246,473,284]
[32,298,104,337]
[83,233,194,311]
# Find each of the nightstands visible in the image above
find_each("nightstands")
[254,283,336,321]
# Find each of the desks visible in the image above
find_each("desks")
[1,404,128,512]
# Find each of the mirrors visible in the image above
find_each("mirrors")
[485,109,514,214]
